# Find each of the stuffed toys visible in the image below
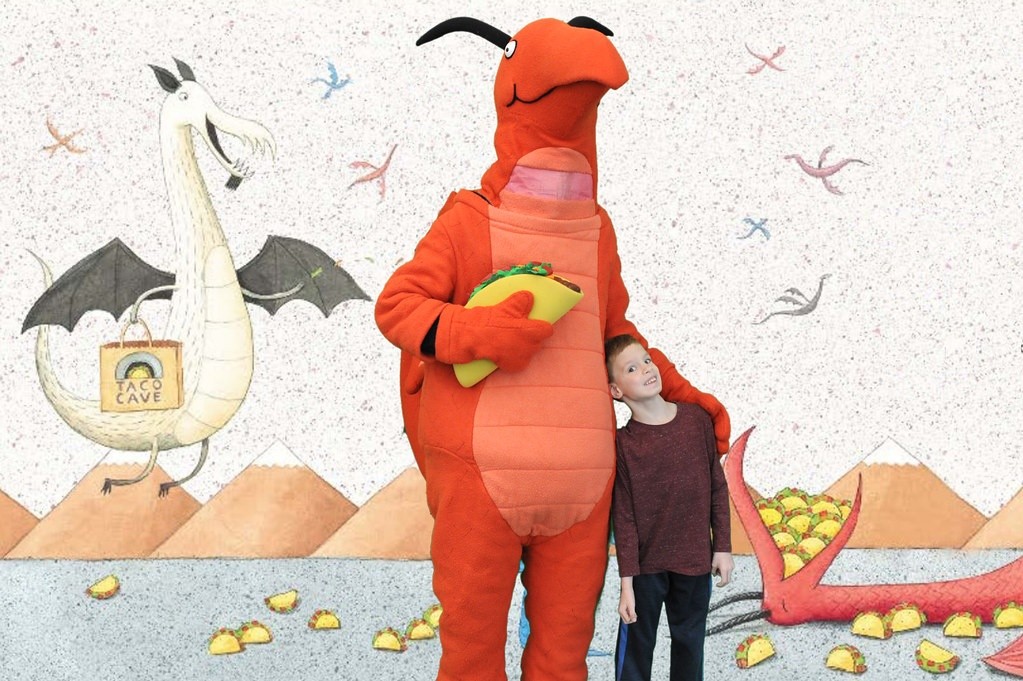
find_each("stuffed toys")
[375,12,731,681]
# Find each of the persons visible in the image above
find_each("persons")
[604,331,734,680]
